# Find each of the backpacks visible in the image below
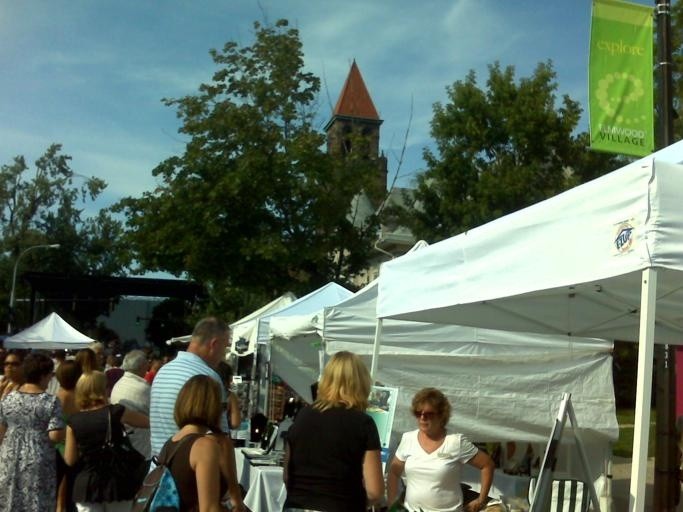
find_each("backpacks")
[132,434,207,511]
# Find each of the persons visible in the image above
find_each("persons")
[0,312,253,511]
[282,351,385,512]
[386,387,496,512]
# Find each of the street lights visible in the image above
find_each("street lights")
[7,243,63,335]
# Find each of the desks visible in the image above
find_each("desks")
[461,462,539,500]
[233,447,288,512]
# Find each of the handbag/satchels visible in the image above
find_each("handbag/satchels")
[96,448,145,502]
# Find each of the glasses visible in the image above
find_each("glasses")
[413,410,440,420]
[2,362,20,367]
